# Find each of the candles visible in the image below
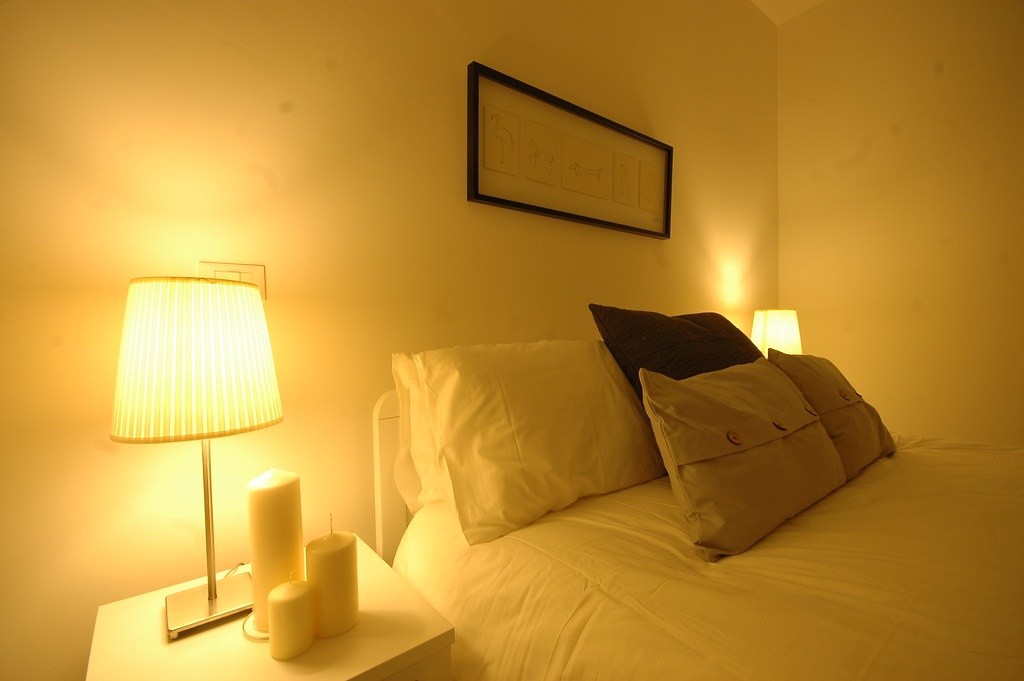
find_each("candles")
[304,513,359,638]
[268,572,314,661]
[248,469,305,632]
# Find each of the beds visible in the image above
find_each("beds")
[372,390,1024,681]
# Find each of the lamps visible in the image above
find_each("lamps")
[111,277,284,639]
[751,309,803,359]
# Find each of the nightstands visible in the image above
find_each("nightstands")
[84,533,454,681]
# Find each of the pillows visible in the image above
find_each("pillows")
[639,356,847,563]
[389,339,668,545]
[768,348,897,481]
[588,303,766,404]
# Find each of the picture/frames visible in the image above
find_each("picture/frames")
[467,61,674,240]
